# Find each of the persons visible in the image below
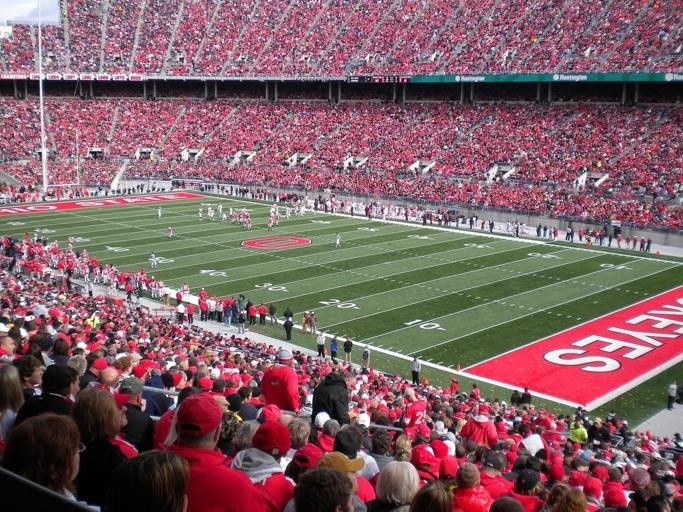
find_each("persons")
[2,233,683,511]
[2,94,683,251]
[1,1,683,76]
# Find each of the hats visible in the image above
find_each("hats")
[260,405,282,421]
[318,452,364,473]
[479,405,489,412]
[507,441,627,508]
[92,358,252,391]
[315,412,330,428]
[292,443,321,469]
[177,393,222,436]
[253,421,291,455]
[405,424,458,478]
[594,417,601,424]
[279,349,293,363]
